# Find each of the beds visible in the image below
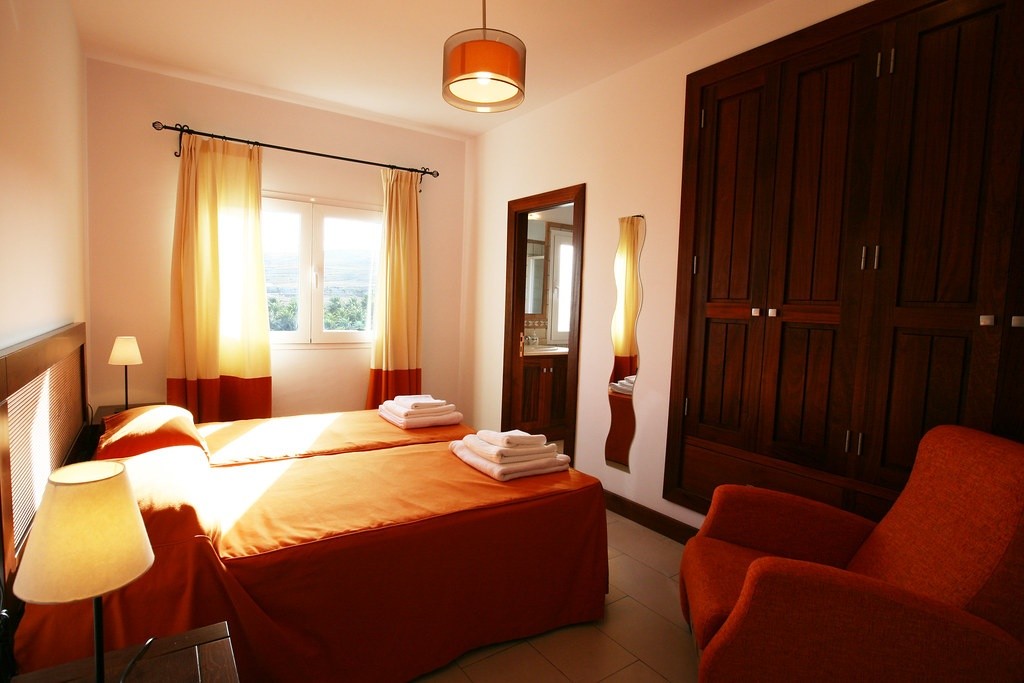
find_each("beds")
[0,321,610,683]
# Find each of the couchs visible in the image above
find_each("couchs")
[679,424,1024,683]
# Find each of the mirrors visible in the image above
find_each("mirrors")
[525,243,545,315]
[605,213,647,474]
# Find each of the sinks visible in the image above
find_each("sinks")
[524,344,569,356]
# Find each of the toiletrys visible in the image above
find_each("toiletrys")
[530,330,539,346]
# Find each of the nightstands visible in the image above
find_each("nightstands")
[10,620,240,683]
[91,402,165,425]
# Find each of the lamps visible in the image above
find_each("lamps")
[108,335,143,413]
[12,461,155,683]
[442,0,527,112]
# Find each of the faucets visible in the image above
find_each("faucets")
[524,335,530,343]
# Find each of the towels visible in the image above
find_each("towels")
[376,393,465,430]
[610,372,635,396]
[448,427,570,482]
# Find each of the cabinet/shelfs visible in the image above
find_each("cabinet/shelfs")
[522,355,568,442]
